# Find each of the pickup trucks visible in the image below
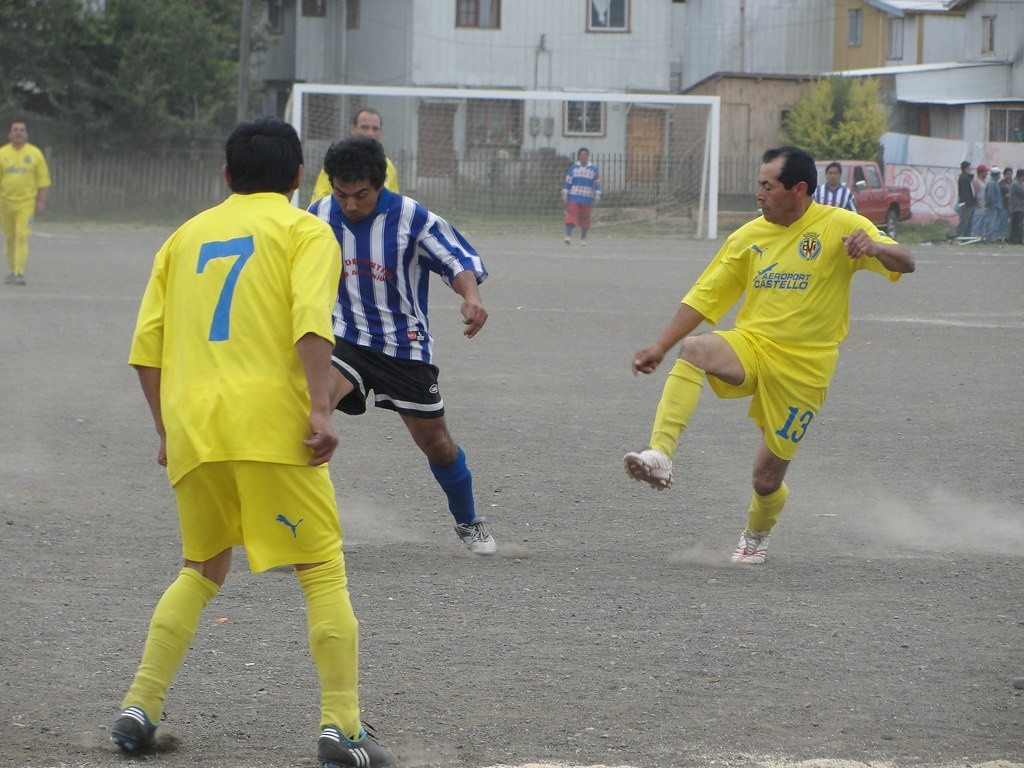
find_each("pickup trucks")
[813,160,912,241]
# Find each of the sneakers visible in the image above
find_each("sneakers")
[730,528,773,565]
[454,513,497,557]
[563,236,571,245]
[110,707,158,754]
[317,726,397,767]
[580,238,588,246]
[5,274,26,285]
[621,450,675,492]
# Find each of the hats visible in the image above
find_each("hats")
[976,165,990,172]
[990,167,1002,174]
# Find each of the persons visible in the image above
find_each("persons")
[955,160,1024,245]
[811,162,858,214]
[310,108,400,205]
[561,148,602,246]
[0,118,52,287]
[622,146,916,567]
[305,134,497,556]
[109,117,399,768]
